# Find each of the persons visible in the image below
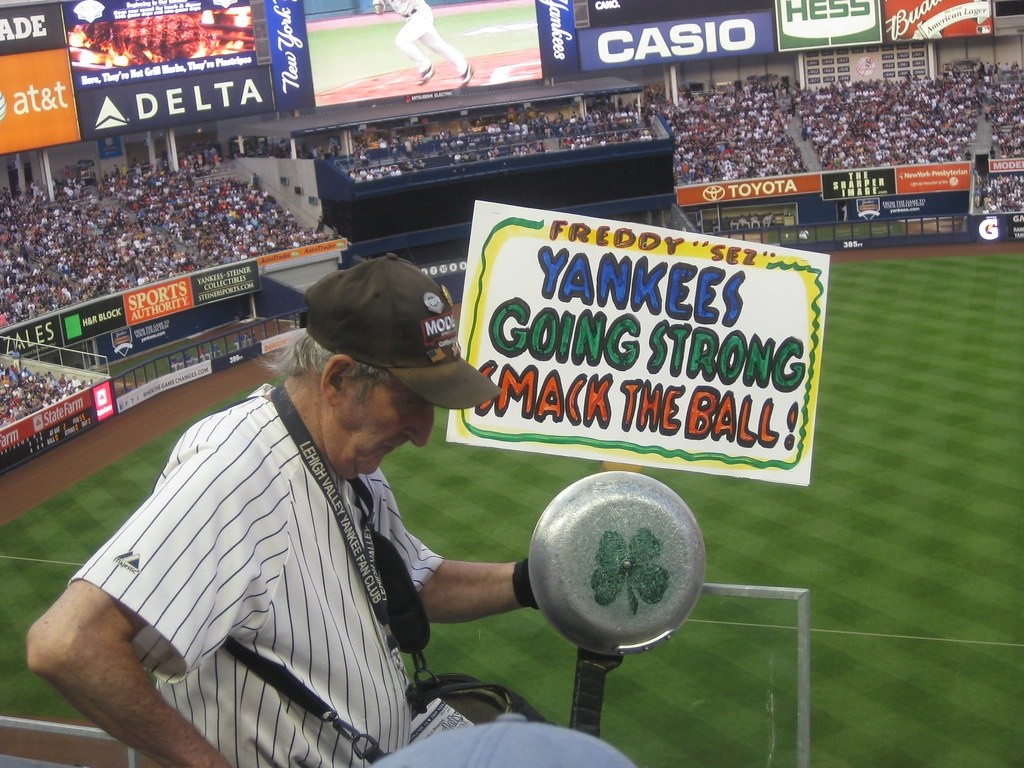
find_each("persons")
[653,72,808,188]
[347,94,659,185]
[373,0,473,87]
[0,143,346,330]
[791,69,981,169]
[713,212,776,232]
[975,173,1024,213]
[952,61,1024,159]
[0,363,94,430]
[169,329,258,372]
[30,252,539,768]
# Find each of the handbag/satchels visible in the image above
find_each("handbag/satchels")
[405,673,557,724]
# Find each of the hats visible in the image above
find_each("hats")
[303,252,501,409]
